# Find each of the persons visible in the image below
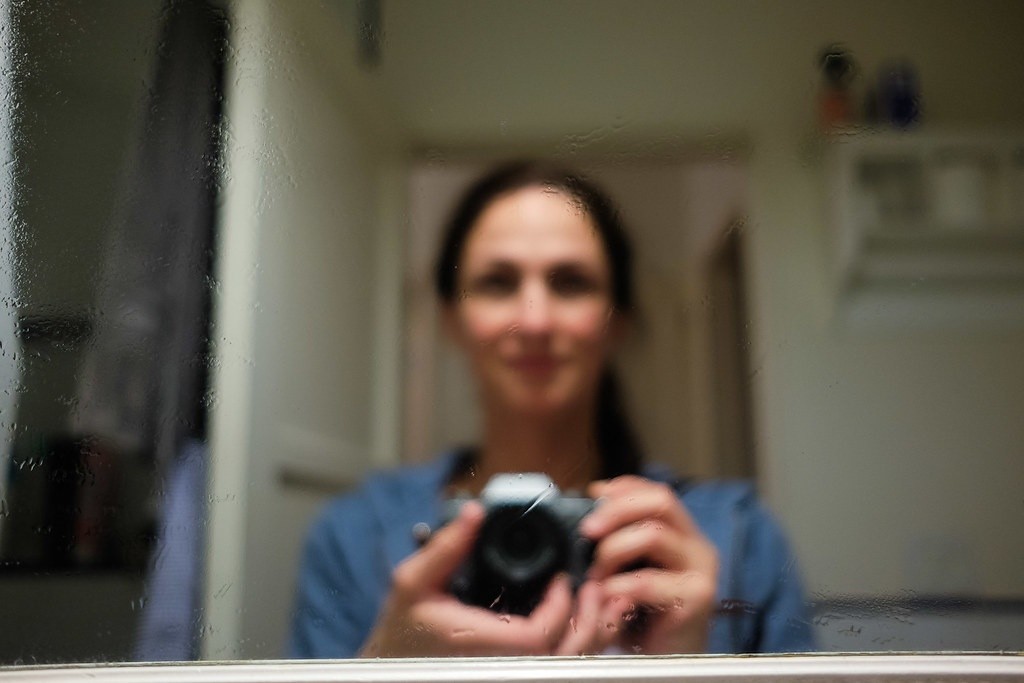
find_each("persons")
[291,159,815,660]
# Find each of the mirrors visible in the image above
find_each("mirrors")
[0,0,1024,683]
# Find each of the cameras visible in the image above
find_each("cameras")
[439,471,644,623]
